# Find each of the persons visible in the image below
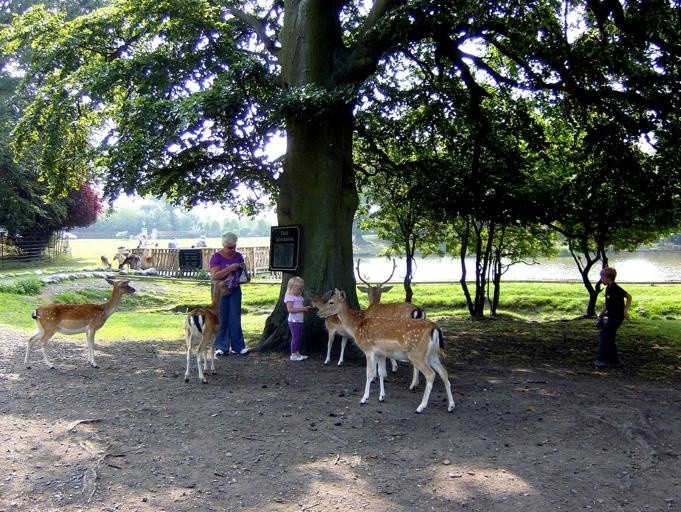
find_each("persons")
[285,276,317,362]
[210,231,251,357]
[151,242,161,263]
[593,268,634,369]
[168,237,178,261]
[197,235,209,251]
[136,234,155,248]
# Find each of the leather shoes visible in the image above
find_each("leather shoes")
[289,351,309,361]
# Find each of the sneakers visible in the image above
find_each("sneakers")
[214,346,250,356]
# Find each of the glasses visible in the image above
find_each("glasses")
[225,243,236,249]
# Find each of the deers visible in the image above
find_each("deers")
[184,277,234,384]
[305,258,456,414]
[101,252,141,269]
[24,279,135,370]
